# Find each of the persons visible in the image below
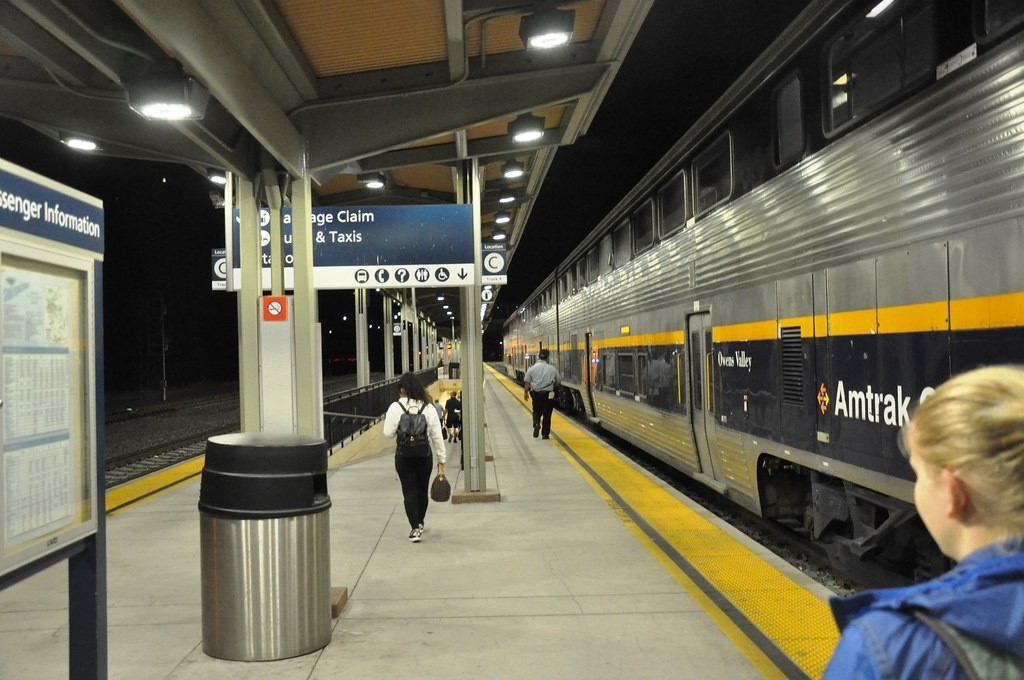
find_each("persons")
[524,349,562,440]
[821,363,1024,680]
[641,346,674,407]
[382,372,447,541]
[433,389,462,443]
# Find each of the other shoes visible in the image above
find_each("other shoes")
[542,434,549,439]
[408,529,422,542]
[448,434,453,443]
[419,523,424,534]
[533,423,540,438]
[454,437,457,443]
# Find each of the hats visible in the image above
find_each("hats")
[539,349,550,358]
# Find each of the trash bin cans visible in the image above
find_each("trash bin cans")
[198,433,333,661]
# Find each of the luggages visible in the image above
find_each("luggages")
[442,427,447,439]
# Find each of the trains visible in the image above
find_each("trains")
[501,0,1024,586]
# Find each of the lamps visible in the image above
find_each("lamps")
[497,190,516,203]
[494,209,511,224]
[446,309,456,320]
[491,229,506,240]
[501,158,524,178]
[507,112,545,143]
[58,132,106,154]
[205,168,227,186]
[435,292,445,301]
[120,76,211,121]
[356,173,386,190]
[519,9,576,51]
[441,304,450,310]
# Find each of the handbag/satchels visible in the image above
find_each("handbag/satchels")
[430,465,453,503]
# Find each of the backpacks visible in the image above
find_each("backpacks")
[394,400,433,460]
